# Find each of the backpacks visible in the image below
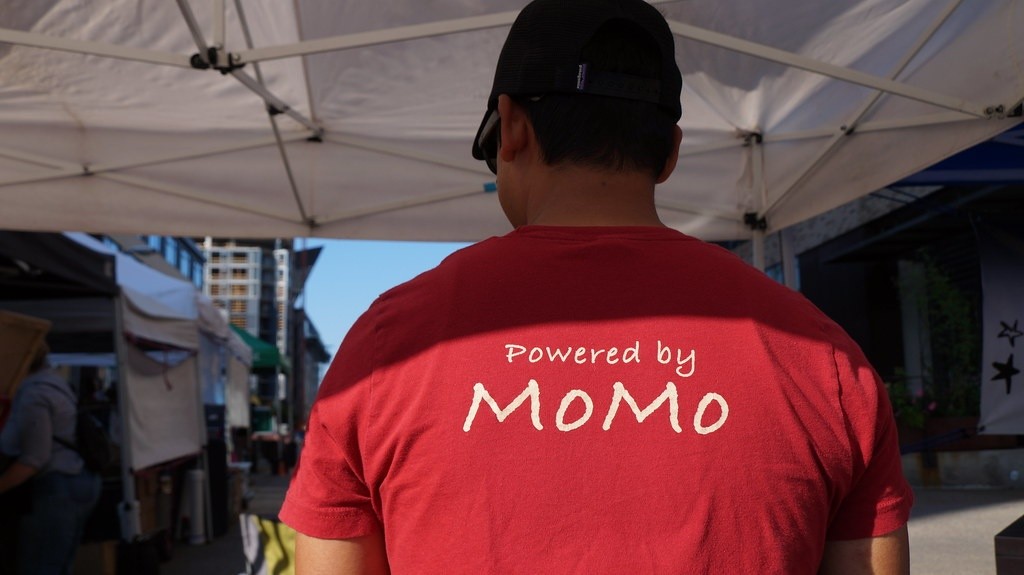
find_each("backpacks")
[19,382,110,471]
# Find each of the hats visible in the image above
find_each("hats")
[472,0,683,160]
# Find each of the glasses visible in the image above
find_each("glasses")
[477,97,539,175]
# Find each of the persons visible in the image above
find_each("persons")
[277,0,916,575]
[0,337,121,575]
